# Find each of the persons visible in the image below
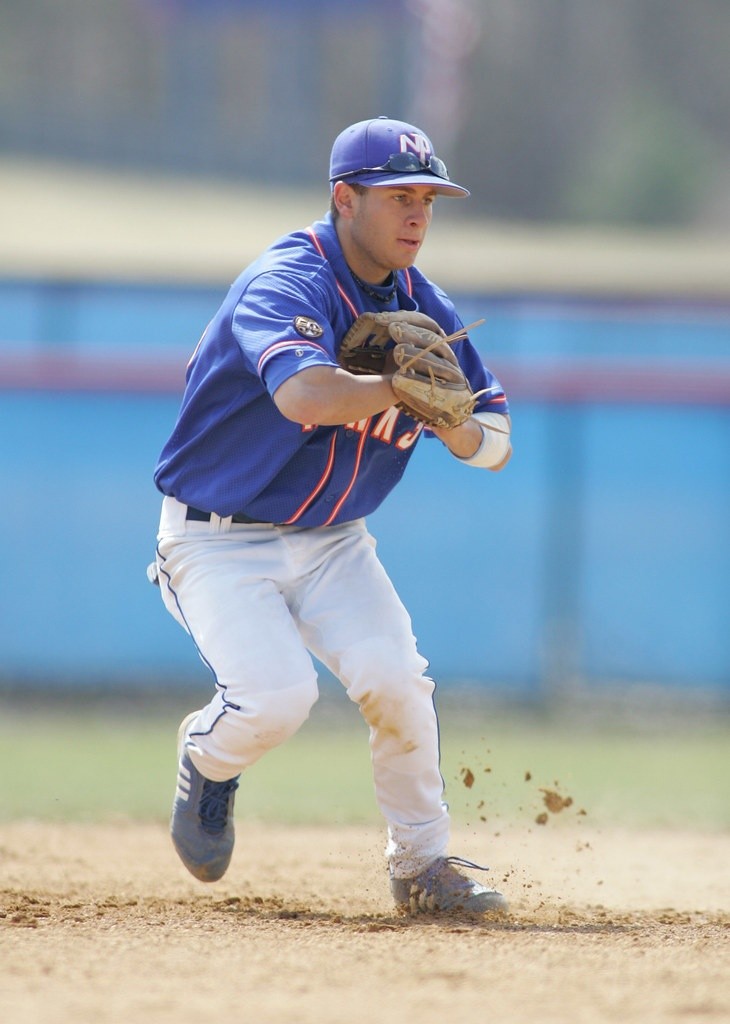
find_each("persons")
[143,115,514,916]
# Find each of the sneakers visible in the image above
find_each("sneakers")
[170,709,241,882]
[387,857,507,918]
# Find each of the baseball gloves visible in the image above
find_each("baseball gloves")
[335,306,480,429]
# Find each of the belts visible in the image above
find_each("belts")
[186,506,272,523]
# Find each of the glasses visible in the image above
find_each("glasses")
[328,151,450,181]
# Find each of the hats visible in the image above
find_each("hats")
[329,116,470,198]
